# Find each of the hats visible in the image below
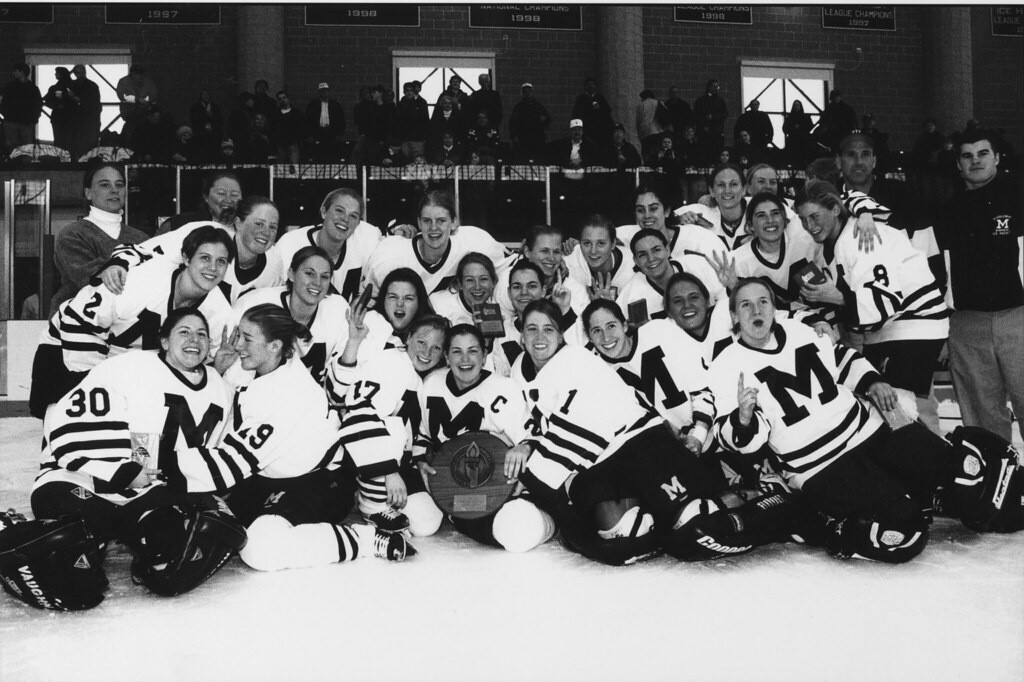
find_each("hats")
[610,122,625,133]
[318,82,329,89]
[570,118,583,129]
[177,126,192,140]
[839,128,875,153]
[220,138,235,149]
[522,83,531,88]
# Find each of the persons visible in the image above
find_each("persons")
[576,298,656,408]
[45,163,153,331]
[838,129,947,304]
[488,161,823,324]
[95,198,285,300]
[510,302,658,565]
[424,252,504,342]
[153,176,243,238]
[692,279,1022,558]
[342,315,448,536]
[268,189,379,298]
[637,273,818,560]
[224,247,354,385]
[3,307,247,613]
[329,267,437,398]
[412,327,552,551]
[794,179,946,436]
[25,221,236,417]
[4,27,865,213]
[355,189,504,303]
[887,131,1023,447]
[226,304,419,570]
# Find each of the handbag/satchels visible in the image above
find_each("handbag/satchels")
[656,99,673,129]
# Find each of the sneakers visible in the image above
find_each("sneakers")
[371,531,417,559]
[363,508,412,540]
